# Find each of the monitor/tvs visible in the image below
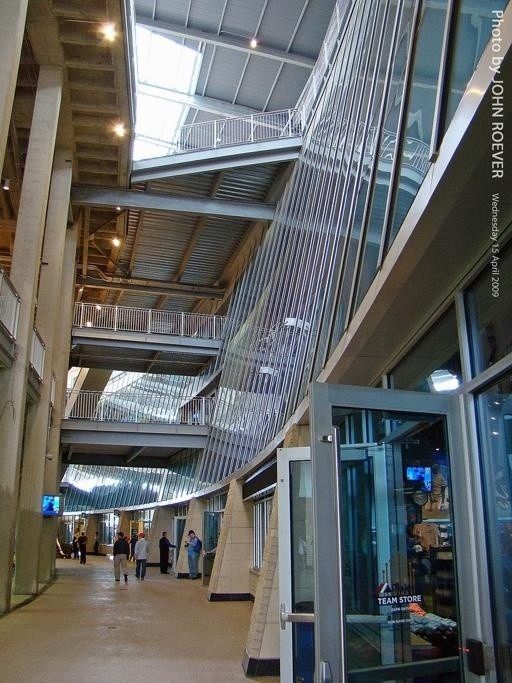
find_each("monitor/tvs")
[406,465,433,493]
[42,495,60,516]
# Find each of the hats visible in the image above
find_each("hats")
[188,530,194,535]
[117,531,124,537]
[138,533,145,537]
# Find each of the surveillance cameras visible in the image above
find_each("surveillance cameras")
[46,454,53,460]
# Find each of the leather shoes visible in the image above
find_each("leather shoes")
[125,574,128,581]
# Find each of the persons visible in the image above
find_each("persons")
[73,535,80,559]
[113,531,130,582]
[135,532,151,580]
[78,531,89,564]
[45,496,56,511]
[184,529,202,579]
[405,525,419,554]
[93,530,100,555]
[206,531,220,554]
[425,463,448,511]
[158,531,179,574]
[113,532,140,563]
